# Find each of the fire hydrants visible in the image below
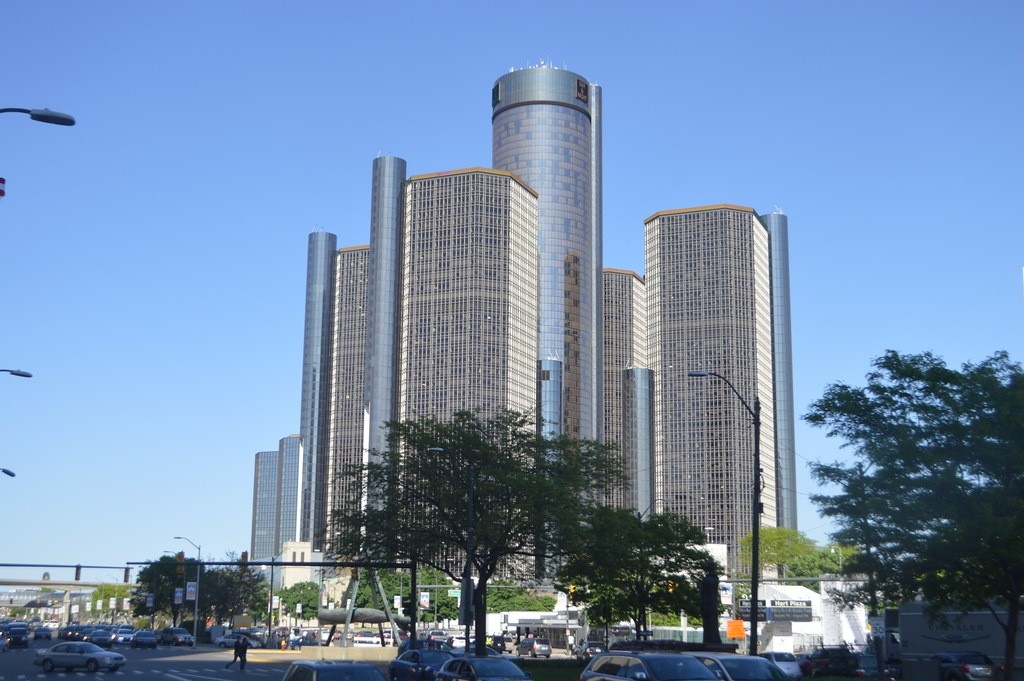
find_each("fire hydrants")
[281,640,287,651]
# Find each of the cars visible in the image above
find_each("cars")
[34,627,53,640]
[517,638,553,657]
[449,644,501,658]
[799,645,903,681]
[389,648,456,681]
[396,637,456,659]
[578,650,726,681]
[484,636,515,654]
[680,652,795,681]
[576,640,609,668]
[248,626,408,651]
[31,641,128,672]
[132,632,158,649]
[57,624,135,649]
[434,656,533,681]
[756,652,803,680]
[282,659,388,681]
[0,618,30,653]
[928,648,1005,681]
[214,634,262,649]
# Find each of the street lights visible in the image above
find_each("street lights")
[269,546,294,650]
[685,370,763,656]
[174,536,202,649]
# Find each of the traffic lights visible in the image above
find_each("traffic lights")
[401,592,411,617]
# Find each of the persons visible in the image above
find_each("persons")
[225,636,246,670]
[448,636,455,646]
[568,632,576,656]
[272,631,278,650]
[239,637,252,672]
[576,637,586,656]
[281,637,288,652]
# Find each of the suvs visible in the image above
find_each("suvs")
[419,630,448,642]
[160,627,195,647]
